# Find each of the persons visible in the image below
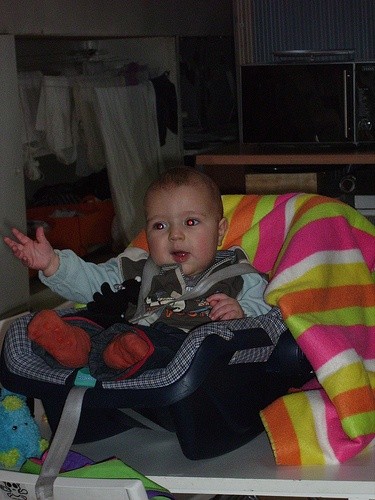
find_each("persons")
[3,166,272,385]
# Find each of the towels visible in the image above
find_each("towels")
[19,70,95,183]
[96,82,167,246]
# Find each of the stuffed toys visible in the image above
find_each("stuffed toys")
[0,387,49,471]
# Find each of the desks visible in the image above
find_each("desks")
[195,142,375,165]
[69,427,375,500]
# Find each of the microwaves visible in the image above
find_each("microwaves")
[239,61,375,144]
